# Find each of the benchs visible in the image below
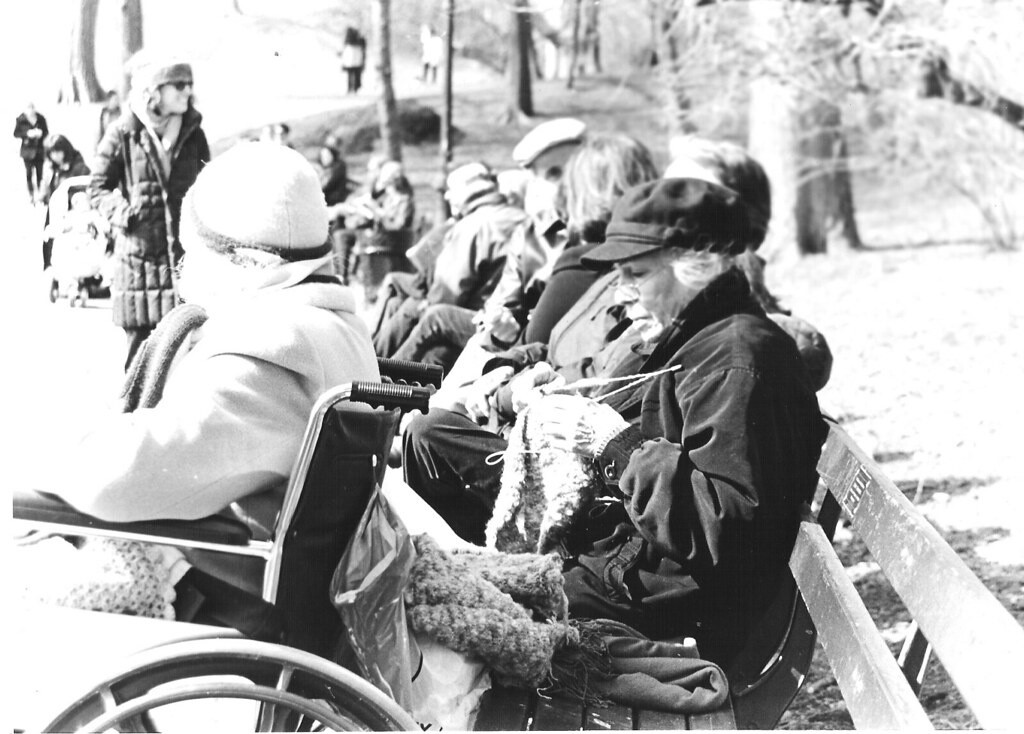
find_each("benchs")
[472,416,1024,731]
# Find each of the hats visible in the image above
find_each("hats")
[511,118,587,169]
[579,177,751,271]
[187,140,333,262]
[457,173,508,217]
[128,40,199,97]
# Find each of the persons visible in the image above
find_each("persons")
[86,50,211,374]
[524,179,827,673]
[14,99,48,200]
[101,90,121,140]
[12,142,381,619]
[40,135,112,298]
[337,26,367,93]
[273,119,833,546]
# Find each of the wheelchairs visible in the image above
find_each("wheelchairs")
[13,356,446,732]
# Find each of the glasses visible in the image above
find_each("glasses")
[161,81,193,91]
[605,258,679,301]
[535,166,566,186]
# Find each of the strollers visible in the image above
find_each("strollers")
[47,175,110,308]
